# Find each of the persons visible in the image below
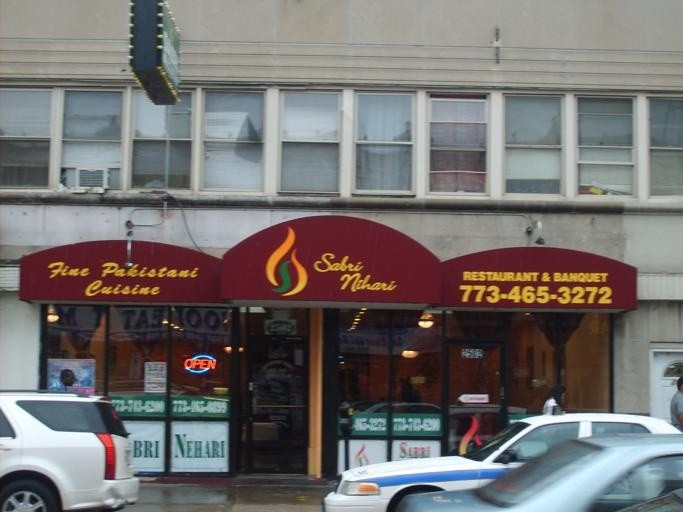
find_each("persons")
[541,385,567,415]
[399,373,422,412]
[338,367,361,401]
[46,369,75,392]
[476,357,492,394]
[670,375,683,432]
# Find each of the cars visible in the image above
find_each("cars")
[363,403,526,450]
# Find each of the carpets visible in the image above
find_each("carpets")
[139,476,333,488]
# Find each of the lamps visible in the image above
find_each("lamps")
[527,219,547,246]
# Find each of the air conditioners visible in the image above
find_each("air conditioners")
[74,168,110,190]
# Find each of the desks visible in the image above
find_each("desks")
[240,439,304,472]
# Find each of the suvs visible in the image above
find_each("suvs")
[0,389,139,512]
